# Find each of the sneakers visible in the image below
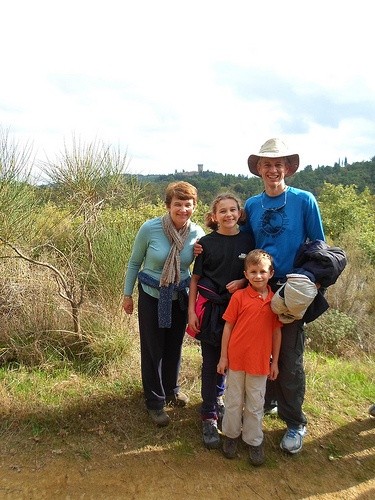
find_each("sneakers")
[280,424,306,454]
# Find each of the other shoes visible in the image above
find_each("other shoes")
[147,407,171,427]
[248,443,265,467]
[223,435,240,460]
[215,395,225,419]
[201,417,222,449]
[166,391,190,406]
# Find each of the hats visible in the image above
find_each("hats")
[248,138,300,178]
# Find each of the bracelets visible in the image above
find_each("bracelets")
[124,296,132,299]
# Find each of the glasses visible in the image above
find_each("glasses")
[261,190,287,212]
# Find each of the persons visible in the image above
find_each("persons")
[216,249,284,465]
[194,138,326,453]
[122,181,207,426]
[188,192,256,448]
